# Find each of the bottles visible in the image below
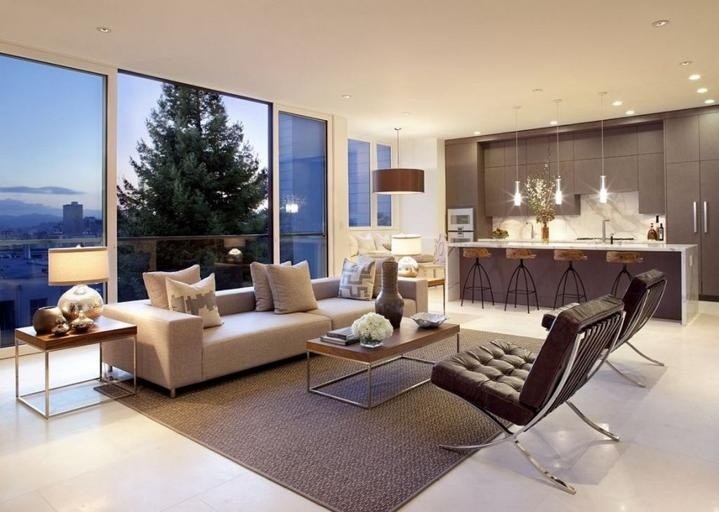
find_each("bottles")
[50,309,94,337]
[647,215,664,241]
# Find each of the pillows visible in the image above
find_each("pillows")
[251,259,292,313]
[165,273,225,328]
[357,233,402,255]
[263,261,317,314]
[339,258,377,301]
[355,255,395,299]
[143,263,200,312]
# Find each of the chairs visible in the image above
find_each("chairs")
[431,294,619,496]
[541,267,668,390]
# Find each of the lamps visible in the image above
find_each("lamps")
[598,92,610,204]
[391,234,426,277]
[48,245,111,321]
[512,106,523,206]
[373,127,425,197]
[552,100,564,204]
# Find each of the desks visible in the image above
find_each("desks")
[424,278,445,315]
[417,262,445,281]
[14,316,139,420]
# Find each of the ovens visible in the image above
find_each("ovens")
[447,207,474,242]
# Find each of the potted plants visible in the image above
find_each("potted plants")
[527,172,558,243]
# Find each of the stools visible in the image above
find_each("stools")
[502,249,540,313]
[456,247,494,309]
[551,250,588,311]
[606,252,642,297]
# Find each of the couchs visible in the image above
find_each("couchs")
[94,271,428,398]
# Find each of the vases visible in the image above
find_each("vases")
[374,261,405,330]
[358,334,385,348]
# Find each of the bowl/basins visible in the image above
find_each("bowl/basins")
[410,311,448,330]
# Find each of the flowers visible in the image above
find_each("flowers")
[349,312,394,341]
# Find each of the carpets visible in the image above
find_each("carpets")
[94,325,547,512]
